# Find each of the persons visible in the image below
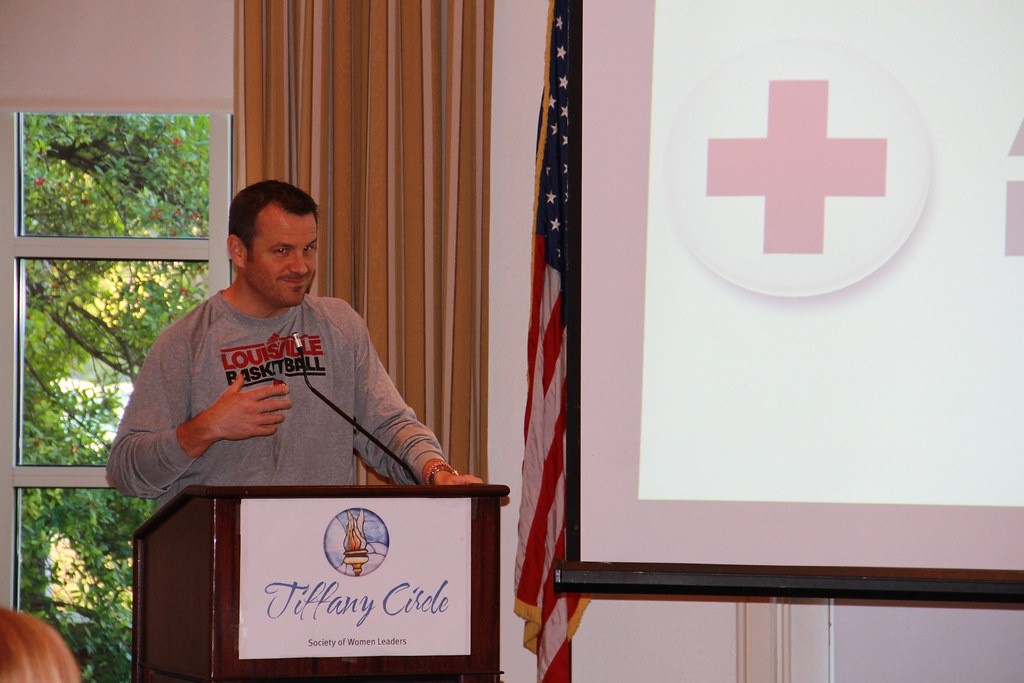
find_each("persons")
[0,604,82,682]
[105,178,485,509]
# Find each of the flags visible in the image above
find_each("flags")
[515,1,590,683]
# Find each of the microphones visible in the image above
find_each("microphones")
[292,332,419,485]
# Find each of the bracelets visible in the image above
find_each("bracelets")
[427,462,458,486]
